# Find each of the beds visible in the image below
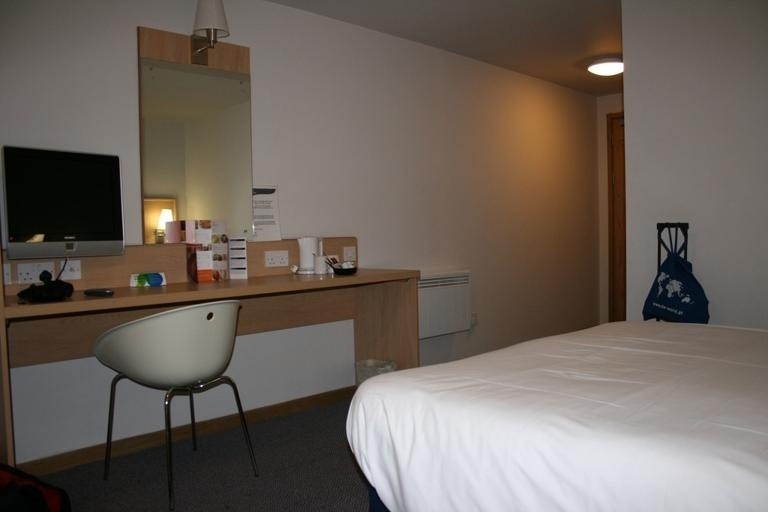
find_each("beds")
[341,316,767,512]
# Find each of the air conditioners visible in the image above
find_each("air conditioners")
[417,269,471,340]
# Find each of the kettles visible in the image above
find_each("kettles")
[289,235,323,275]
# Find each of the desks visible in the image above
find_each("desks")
[0,236,424,474]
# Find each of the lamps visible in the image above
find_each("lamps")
[151,206,176,243]
[187,3,230,66]
[583,55,624,80]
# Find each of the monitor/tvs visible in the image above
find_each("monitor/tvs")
[0,145,124,260]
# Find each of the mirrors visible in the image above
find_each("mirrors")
[137,54,257,245]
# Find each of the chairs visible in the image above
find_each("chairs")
[93,299,260,510]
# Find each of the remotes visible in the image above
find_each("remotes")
[83,288,114,297]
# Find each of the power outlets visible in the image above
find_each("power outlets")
[264,249,291,269]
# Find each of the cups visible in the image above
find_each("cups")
[313,255,338,274]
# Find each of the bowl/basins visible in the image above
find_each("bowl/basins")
[331,263,357,274]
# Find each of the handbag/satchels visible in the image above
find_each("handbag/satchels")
[642,252,710,324]
